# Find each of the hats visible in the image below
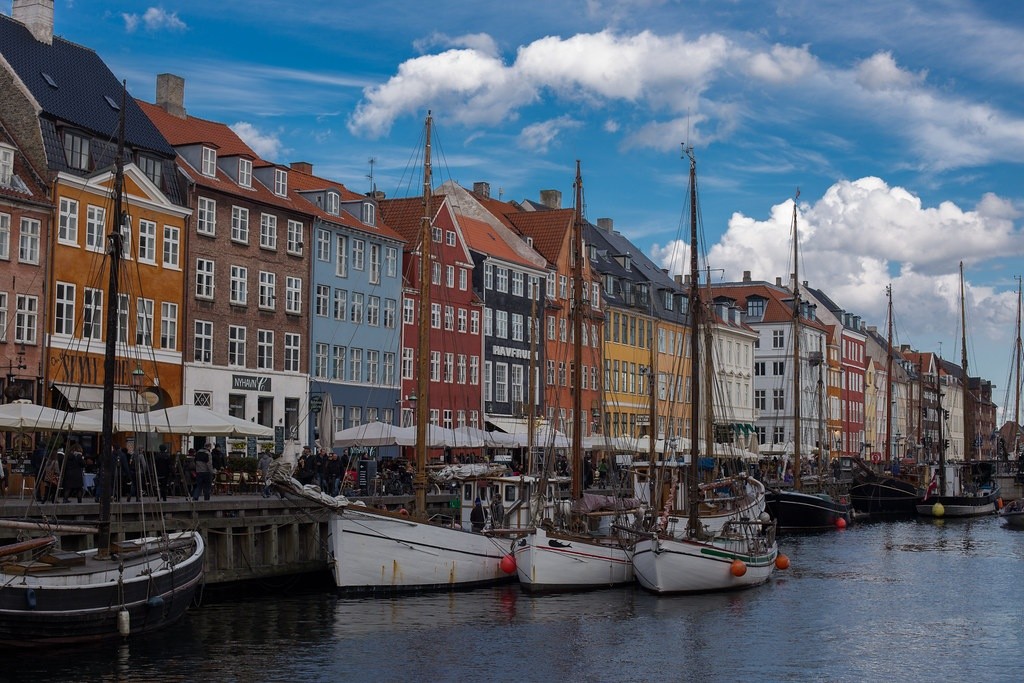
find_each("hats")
[474,498,482,505]
[302,446,312,449]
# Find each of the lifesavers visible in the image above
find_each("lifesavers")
[399,509,409,516]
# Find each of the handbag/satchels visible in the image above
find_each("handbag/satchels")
[45,471,60,485]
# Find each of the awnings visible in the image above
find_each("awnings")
[54,384,150,412]
[490,420,565,437]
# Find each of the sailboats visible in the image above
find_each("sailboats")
[1,81,205,659]
[310,106,1023,595]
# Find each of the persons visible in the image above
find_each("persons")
[491,494,504,529]
[585,456,617,490]
[212,443,227,495]
[452,452,524,476]
[257,452,274,498]
[193,444,214,500]
[555,455,572,489]
[31,435,197,503]
[471,498,488,532]
[723,454,840,482]
[386,457,415,471]
[297,446,352,497]
[0,445,8,499]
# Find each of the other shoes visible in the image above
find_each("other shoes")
[52,500,60,504]
[136,498,141,503]
[36,498,43,502]
[95,497,100,503]
[41,499,46,504]
[78,497,82,503]
[62,498,72,504]
[127,496,132,502]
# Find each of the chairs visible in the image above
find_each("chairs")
[217,471,266,494]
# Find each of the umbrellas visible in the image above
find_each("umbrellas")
[317,422,417,459]
[745,442,820,469]
[509,433,759,464]
[137,404,273,452]
[315,393,335,493]
[402,423,484,447]
[72,409,155,455]
[454,426,519,462]
[0,403,102,454]
